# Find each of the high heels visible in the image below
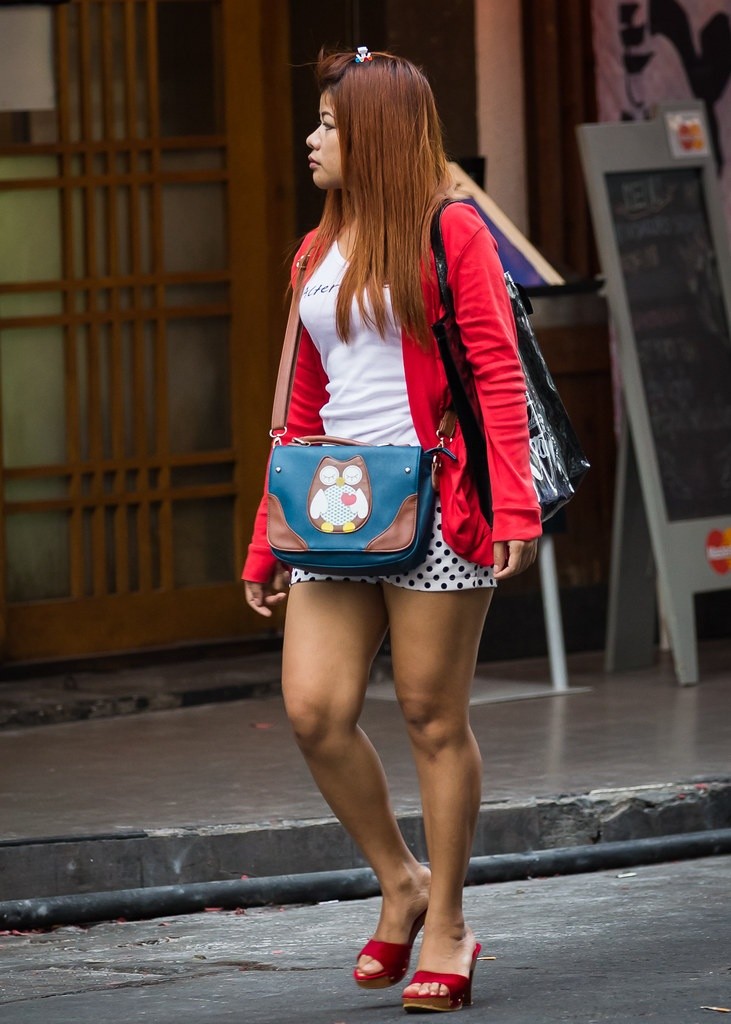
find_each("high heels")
[355,911,429,988]
[405,945,482,1012]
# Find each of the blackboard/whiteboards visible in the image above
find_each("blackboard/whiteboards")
[576,121,731,592]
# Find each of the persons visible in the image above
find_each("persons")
[240,46,542,1012]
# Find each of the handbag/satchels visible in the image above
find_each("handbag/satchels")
[431,197,591,534]
[267,437,440,576]
